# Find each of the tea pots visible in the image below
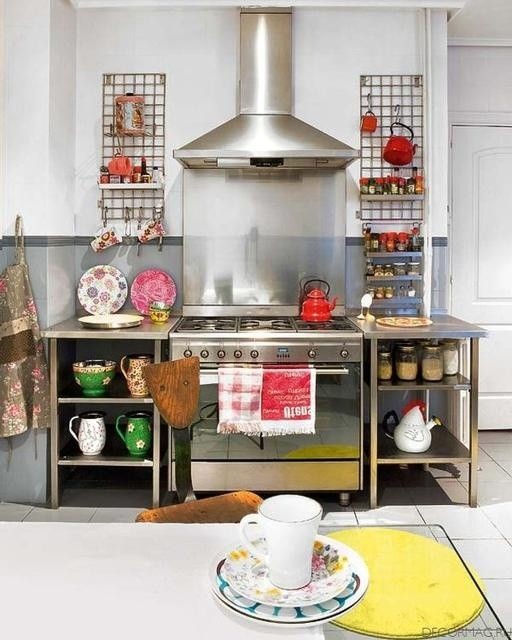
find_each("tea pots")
[299,279,339,322]
[382,120,419,165]
[381,400,441,453]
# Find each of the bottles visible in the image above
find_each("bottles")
[378,340,457,382]
[139,156,150,183]
[150,166,161,183]
[100,166,106,174]
[133,166,141,183]
[110,173,121,183]
[102,169,109,183]
[365,226,421,297]
[360,167,424,194]
[121,175,132,183]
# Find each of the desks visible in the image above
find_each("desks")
[0,521,509,640]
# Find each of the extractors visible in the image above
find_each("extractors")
[172,5,361,174]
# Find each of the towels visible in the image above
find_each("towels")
[215,368,316,437]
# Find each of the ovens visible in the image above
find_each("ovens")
[168,362,361,462]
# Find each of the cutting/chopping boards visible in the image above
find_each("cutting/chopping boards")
[140,356,200,429]
[78,315,144,328]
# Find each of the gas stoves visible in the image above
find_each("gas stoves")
[176,317,357,332]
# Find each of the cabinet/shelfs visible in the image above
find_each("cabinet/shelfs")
[345,314,488,509]
[366,250,423,316]
[40,315,181,509]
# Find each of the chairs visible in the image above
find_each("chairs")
[135,491,265,523]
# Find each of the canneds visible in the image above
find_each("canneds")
[393,262,406,275]
[394,343,418,381]
[377,351,393,380]
[421,344,443,381]
[406,262,420,275]
[438,340,459,376]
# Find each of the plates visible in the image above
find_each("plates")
[376,317,433,327]
[207,535,370,629]
[76,263,128,314]
[129,269,177,316]
[224,538,352,606]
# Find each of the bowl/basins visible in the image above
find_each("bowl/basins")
[149,310,170,324]
[150,299,172,313]
[71,358,116,396]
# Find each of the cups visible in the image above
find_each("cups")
[89,226,122,253]
[119,352,151,397]
[106,153,131,177]
[67,411,107,457]
[136,217,166,244]
[114,409,151,458]
[239,493,323,591]
[361,111,378,131]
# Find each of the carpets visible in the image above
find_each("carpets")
[322,530,487,639]
